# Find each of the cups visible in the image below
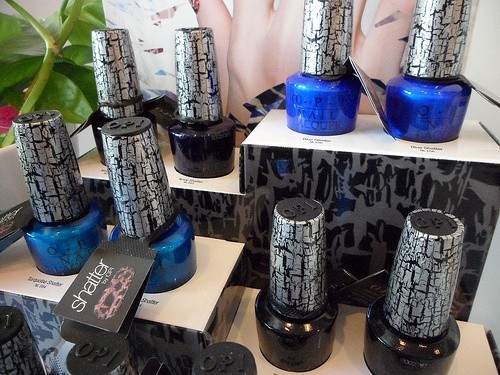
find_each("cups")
[1,0,472,375]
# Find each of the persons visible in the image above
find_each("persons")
[189,0,417,149]
